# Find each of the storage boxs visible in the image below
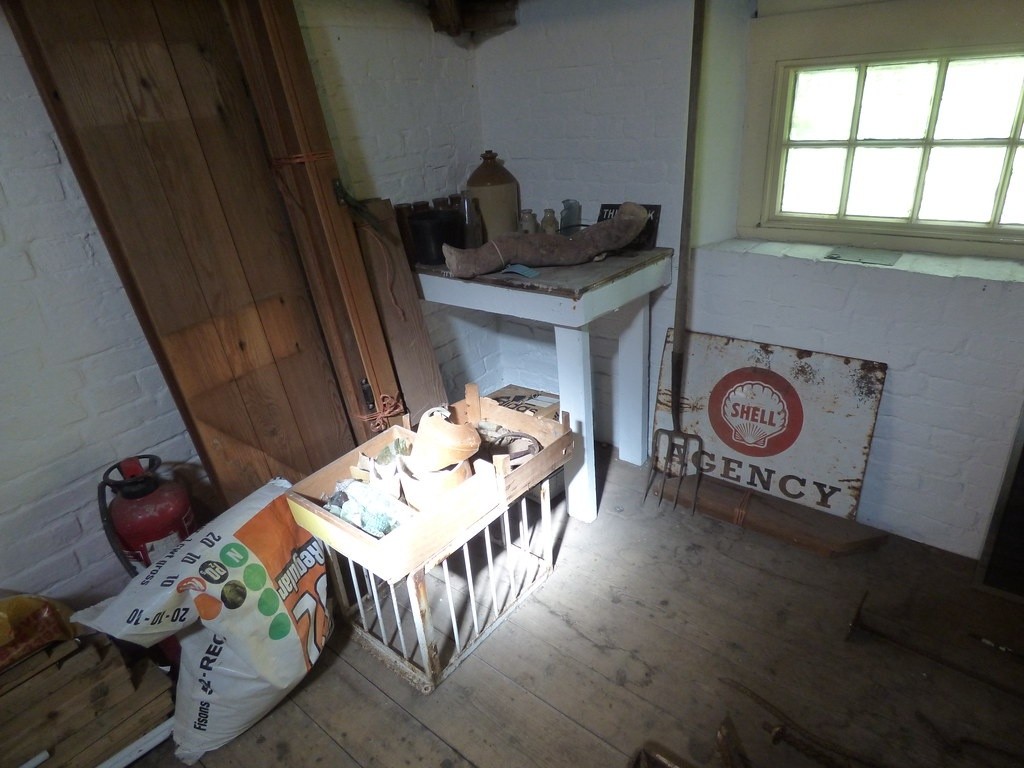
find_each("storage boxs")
[284,383,575,585]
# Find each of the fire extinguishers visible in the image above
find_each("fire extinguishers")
[96,454,198,579]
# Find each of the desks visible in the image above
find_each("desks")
[411,245,676,525]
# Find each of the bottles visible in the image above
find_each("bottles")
[518,199,583,237]
[395,188,484,267]
